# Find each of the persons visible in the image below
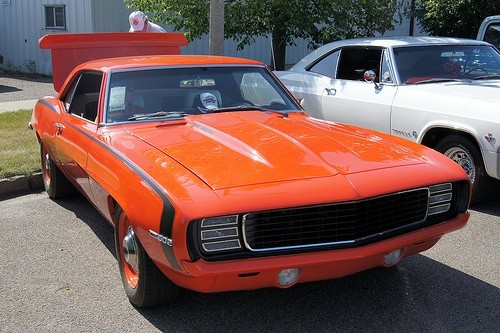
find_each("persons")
[95,77,148,124]
[194,71,254,108]
[128,11,167,33]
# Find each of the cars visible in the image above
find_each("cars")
[476,16,500,52]
[240,33,499,204]
[27,27,472,312]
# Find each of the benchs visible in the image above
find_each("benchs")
[73,88,188,115]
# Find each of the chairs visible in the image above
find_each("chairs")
[182,89,222,111]
[83,99,136,123]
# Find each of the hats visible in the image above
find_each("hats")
[128,10,147,31]
